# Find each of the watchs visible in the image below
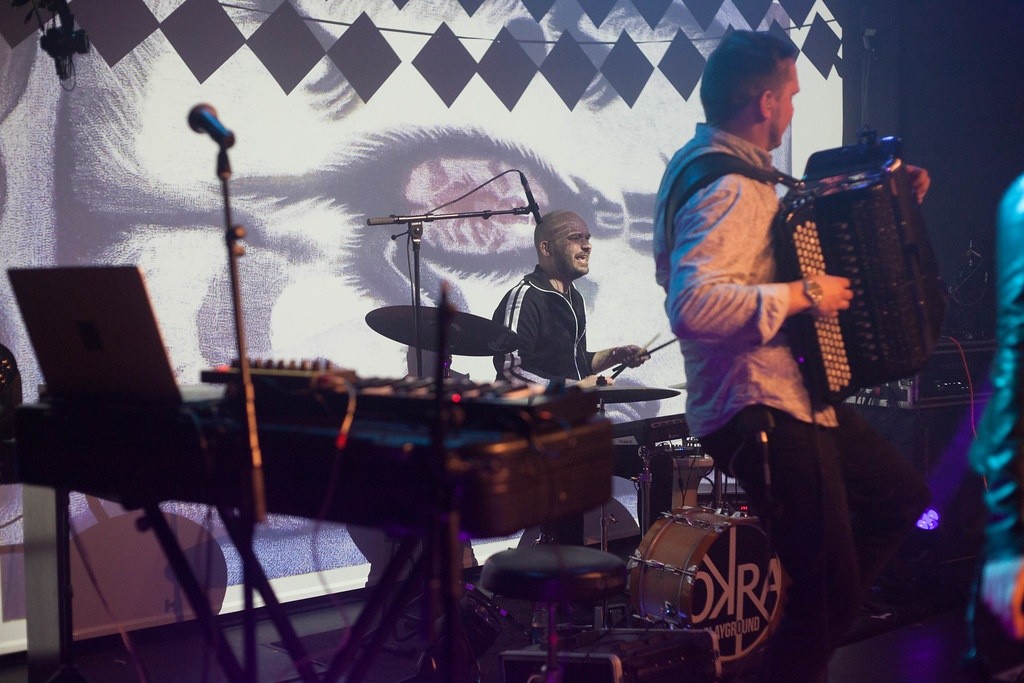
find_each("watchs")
[612,347,622,364]
[802,277,824,312]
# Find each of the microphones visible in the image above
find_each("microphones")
[520,173,542,225]
[188,103,235,148]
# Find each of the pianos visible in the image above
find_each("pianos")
[0,360,614,538]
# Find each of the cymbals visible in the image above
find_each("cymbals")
[365,305,521,357]
[584,385,682,404]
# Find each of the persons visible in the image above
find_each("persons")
[651,22,933,683]
[491,207,650,547]
[968,169,1024,682]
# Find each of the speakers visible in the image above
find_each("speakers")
[518,496,641,565]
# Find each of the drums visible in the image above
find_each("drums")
[628,504,787,662]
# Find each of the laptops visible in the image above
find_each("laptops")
[7,264,225,409]
